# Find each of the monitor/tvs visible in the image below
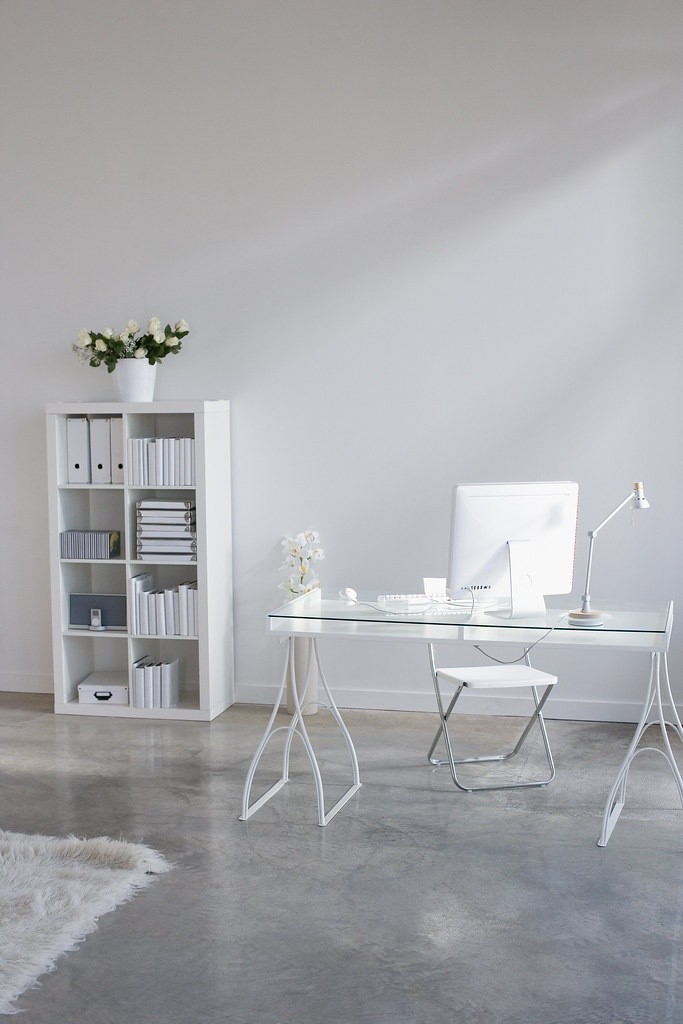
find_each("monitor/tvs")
[446,481,579,619]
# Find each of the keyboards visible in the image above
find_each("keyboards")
[378,593,498,605]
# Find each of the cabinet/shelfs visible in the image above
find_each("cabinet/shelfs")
[43,399,232,722]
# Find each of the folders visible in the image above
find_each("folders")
[89,419,112,484]
[110,417,124,484]
[65,416,90,484]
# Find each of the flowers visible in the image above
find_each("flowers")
[69,316,190,373]
[277,525,323,605]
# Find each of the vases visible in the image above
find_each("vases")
[115,356,156,402]
[287,636,317,715]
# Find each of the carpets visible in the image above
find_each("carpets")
[0,829,174,1015]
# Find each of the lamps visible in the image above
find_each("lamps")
[567,481,650,628]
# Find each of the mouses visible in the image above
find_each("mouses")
[338,588,358,601]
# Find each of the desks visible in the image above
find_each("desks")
[238,588,683,847]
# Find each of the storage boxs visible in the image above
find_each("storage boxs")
[76,671,129,705]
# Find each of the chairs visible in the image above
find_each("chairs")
[423,577,558,791]
